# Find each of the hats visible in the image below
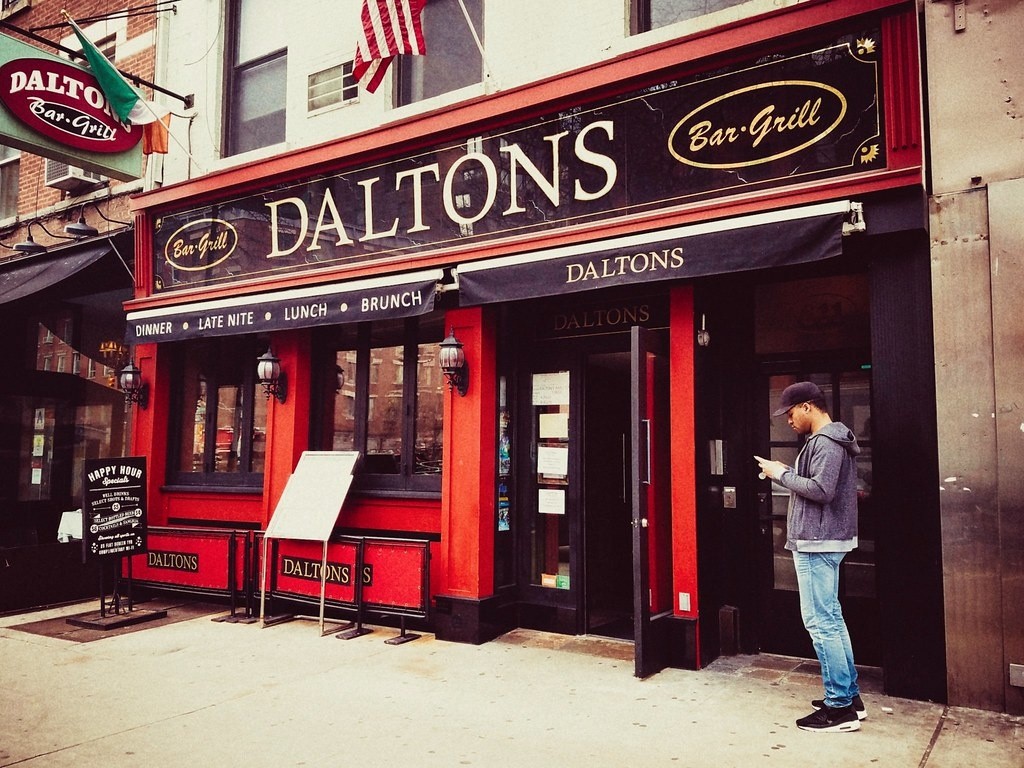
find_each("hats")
[773,382,823,416]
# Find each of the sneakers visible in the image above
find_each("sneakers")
[796,704,860,732]
[812,694,867,720]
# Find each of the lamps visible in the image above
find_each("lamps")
[438,324,471,397]
[64,202,136,237]
[195,377,207,413]
[256,349,286,404]
[336,364,346,395]
[120,356,149,413]
[13,221,80,251]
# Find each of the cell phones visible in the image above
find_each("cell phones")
[754,456,765,464]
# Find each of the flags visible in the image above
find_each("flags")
[64,11,171,127]
[352,0,429,99]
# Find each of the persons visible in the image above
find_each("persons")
[754,381,868,736]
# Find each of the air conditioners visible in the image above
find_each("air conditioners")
[44,158,108,193]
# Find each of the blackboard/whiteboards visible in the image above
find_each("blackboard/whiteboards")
[263,450,362,542]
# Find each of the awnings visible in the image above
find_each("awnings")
[0,245,135,325]
